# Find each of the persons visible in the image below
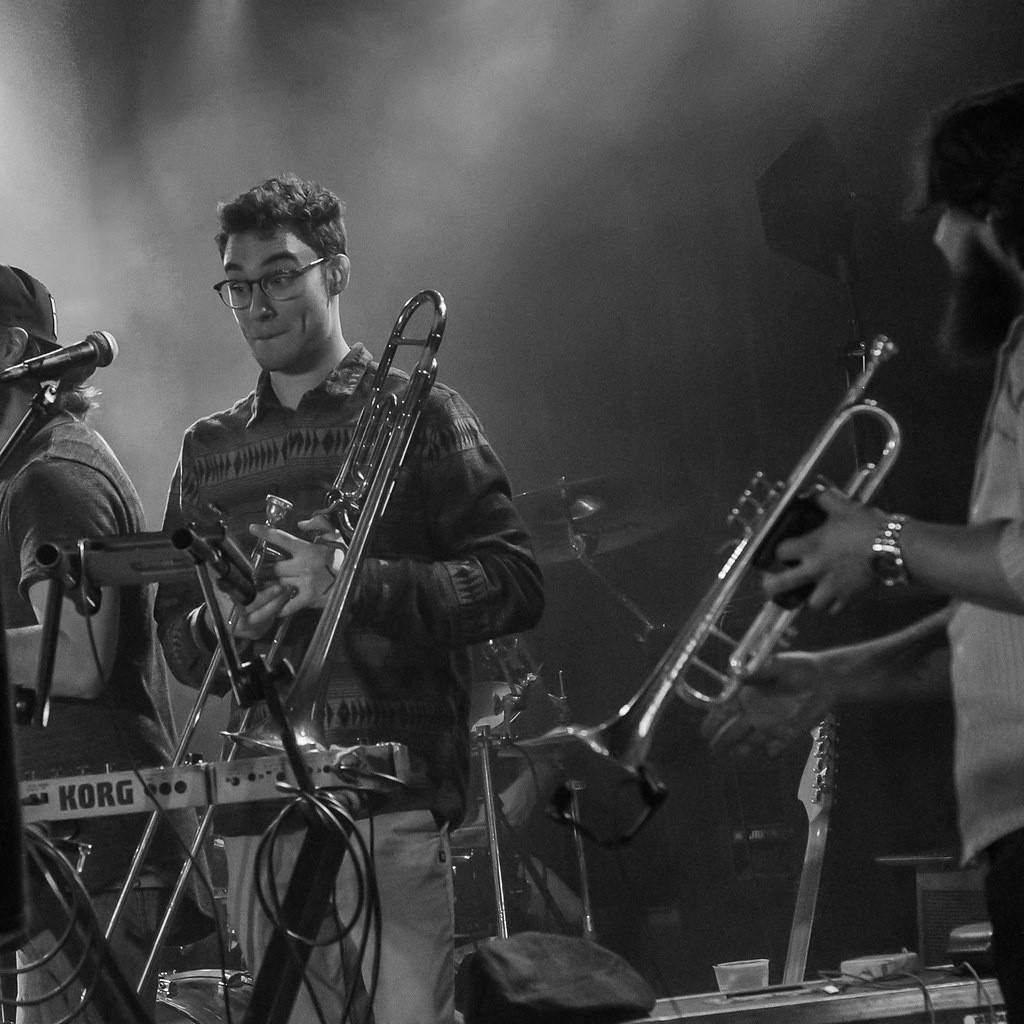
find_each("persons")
[0,264,217,1024]
[702,85,1024,1024]
[151,173,544,1024]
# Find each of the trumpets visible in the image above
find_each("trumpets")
[562,331,902,803]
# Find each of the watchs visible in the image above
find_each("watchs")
[869,511,907,591]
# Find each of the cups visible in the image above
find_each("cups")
[712,959,770,993]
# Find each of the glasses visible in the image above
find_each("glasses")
[212,255,333,309]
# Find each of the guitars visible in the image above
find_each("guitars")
[781,708,844,988]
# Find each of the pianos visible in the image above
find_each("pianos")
[19,740,436,826]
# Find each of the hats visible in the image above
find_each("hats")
[918,78,1024,215]
[0,265,64,348]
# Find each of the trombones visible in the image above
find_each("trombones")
[71,287,451,1024]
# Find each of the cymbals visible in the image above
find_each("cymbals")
[509,463,627,521]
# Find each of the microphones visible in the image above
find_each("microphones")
[546,785,573,820]
[0,330,119,385]
